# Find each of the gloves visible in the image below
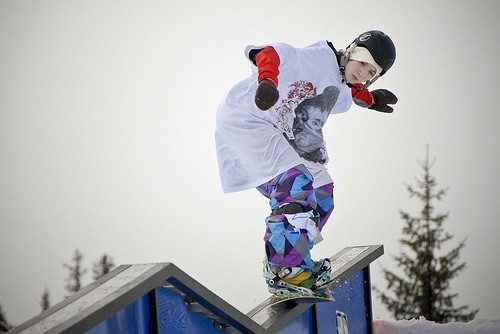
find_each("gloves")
[254,79,281,111]
[369,89,398,113]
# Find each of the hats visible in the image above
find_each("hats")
[346,30,396,76]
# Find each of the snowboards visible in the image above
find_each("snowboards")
[270,283,336,308]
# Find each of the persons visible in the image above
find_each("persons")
[215,29,398,295]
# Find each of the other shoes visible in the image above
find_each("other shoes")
[264,258,333,297]
